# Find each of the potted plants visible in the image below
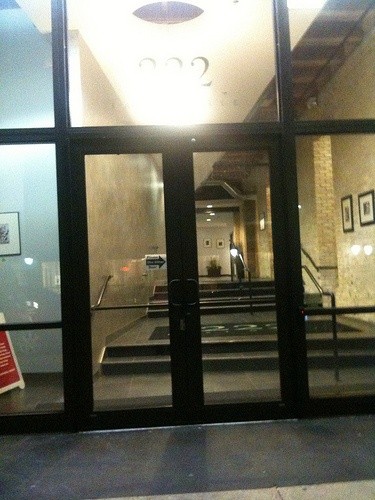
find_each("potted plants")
[207,258,221,276]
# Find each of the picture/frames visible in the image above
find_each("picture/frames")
[216,238,225,249]
[341,194,354,233]
[0,211,22,257]
[358,189,375,227]
[203,238,212,248]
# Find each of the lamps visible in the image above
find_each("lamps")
[133,0,212,124]
